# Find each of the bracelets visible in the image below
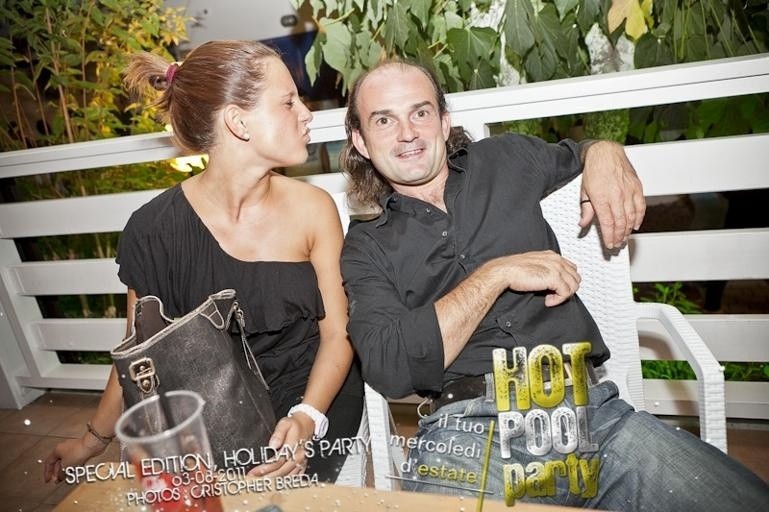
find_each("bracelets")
[86,416,113,446]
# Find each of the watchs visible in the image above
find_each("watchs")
[288,403,329,442]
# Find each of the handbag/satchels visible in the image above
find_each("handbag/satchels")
[109,287,278,477]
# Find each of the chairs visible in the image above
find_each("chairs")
[365,154,727,493]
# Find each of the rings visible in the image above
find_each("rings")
[579,199,591,204]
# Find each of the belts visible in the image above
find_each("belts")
[429,359,597,414]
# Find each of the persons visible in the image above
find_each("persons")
[340,54,768,512]
[42,34,365,481]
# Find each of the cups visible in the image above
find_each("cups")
[115,390,223,512]
